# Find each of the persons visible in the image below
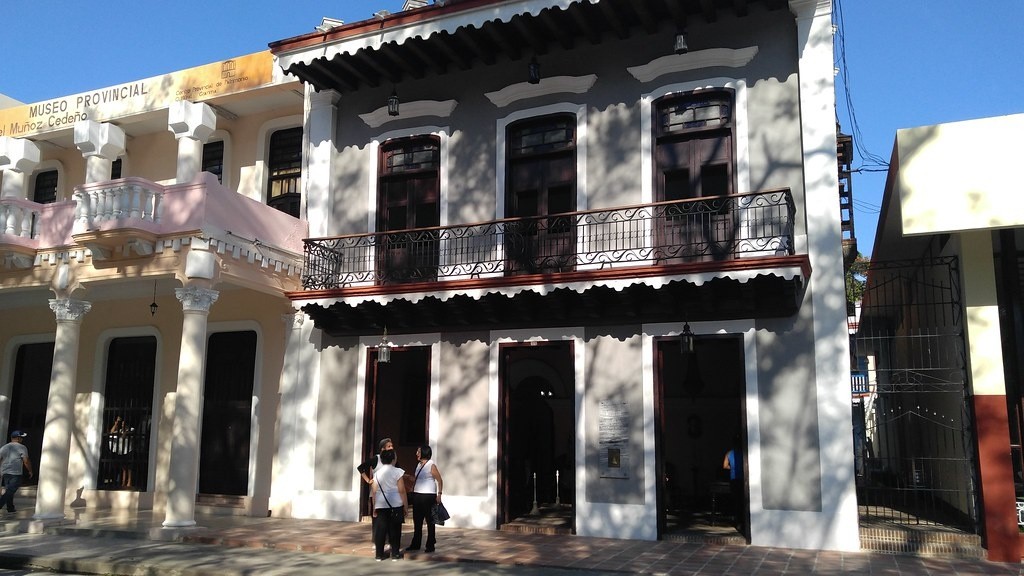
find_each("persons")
[109,421,134,486]
[356,439,408,561]
[722,450,740,530]
[405,445,443,553]
[0,431,33,512]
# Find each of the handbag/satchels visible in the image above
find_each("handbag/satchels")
[391,507,405,523]
[406,492,414,505]
[438,503,450,521]
[431,504,444,526]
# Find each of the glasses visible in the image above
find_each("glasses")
[417,451,421,454]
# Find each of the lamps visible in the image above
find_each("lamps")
[847,302,856,316]
[384,83,400,117]
[674,22,687,55]
[679,322,697,358]
[528,50,540,84]
[375,324,398,367]
[150,280,158,317]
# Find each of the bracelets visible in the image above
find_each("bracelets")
[438,492,442,494]
[367,481,370,482]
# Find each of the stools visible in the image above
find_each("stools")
[706,481,739,524]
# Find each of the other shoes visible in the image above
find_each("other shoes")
[391,551,404,562]
[425,547,435,553]
[375,553,390,562]
[406,545,420,551]
